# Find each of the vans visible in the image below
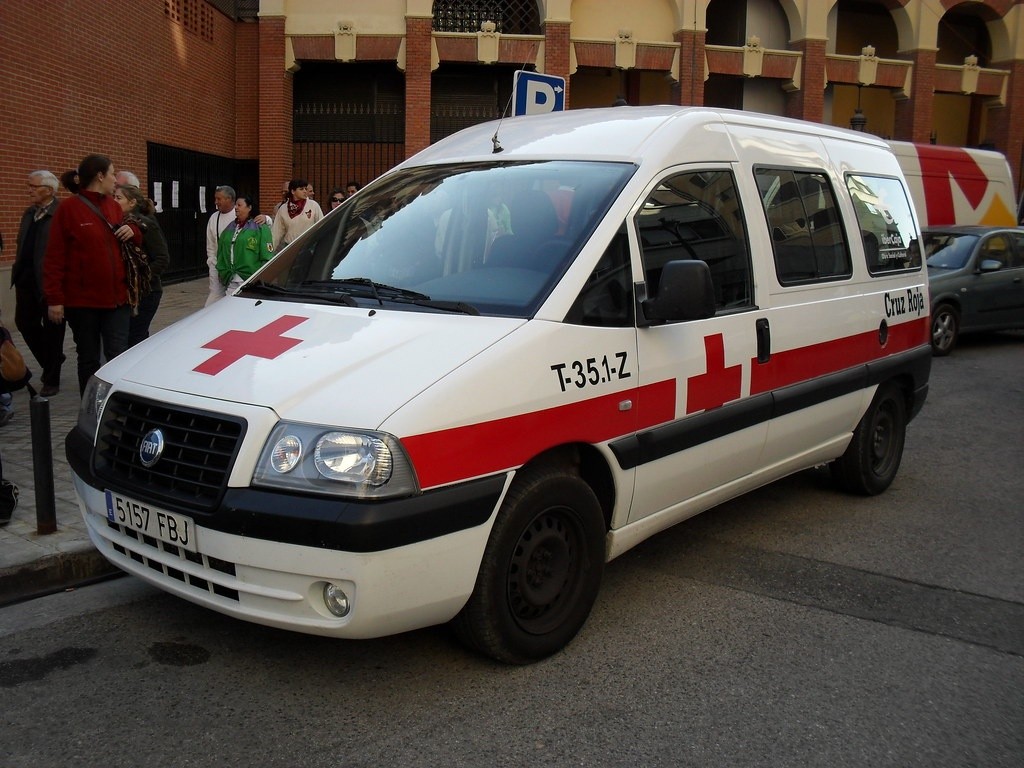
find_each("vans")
[878,139,1018,232]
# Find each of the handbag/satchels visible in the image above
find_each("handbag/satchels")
[0,323,37,398]
[122,239,153,315]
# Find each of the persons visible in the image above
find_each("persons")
[204,177,362,311]
[0,315,32,523]
[9,154,167,398]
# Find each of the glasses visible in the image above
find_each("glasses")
[27,183,52,190]
[331,197,346,203]
[216,186,227,190]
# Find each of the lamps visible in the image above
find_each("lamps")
[849,82,868,131]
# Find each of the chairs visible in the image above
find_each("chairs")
[488,190,558,271]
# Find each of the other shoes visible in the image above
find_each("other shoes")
[40,383,59,396]
[0,400,14,428]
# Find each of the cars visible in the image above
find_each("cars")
[921,226,1024,360]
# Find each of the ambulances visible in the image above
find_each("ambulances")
[63,106,935,668]
